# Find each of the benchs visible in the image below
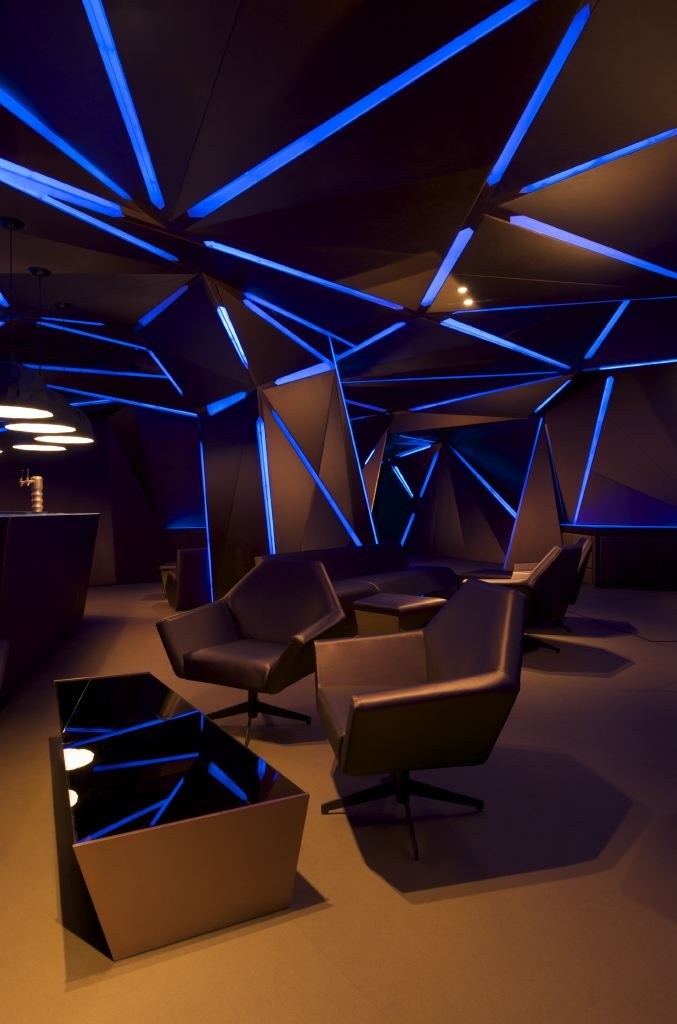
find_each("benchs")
[255,541,459,639]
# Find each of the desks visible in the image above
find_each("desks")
[52,671,310,961]
[462,570,512,579]
[560,523,677,587]
[353,593,447,635]
[166,510,207,534]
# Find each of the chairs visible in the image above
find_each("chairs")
[461,537,593,656]
[155,555,346,746]
[314,580,527,861]
[161,548,211,610]
